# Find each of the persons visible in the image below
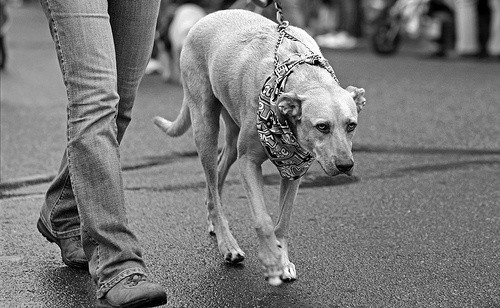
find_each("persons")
[37,0,168,308]
[143,0,500,77]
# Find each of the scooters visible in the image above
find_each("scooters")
[369,1,454,56]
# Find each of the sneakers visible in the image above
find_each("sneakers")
[37,217,88,268]
[105,274,167,308]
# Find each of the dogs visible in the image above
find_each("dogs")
[152,8,359,286]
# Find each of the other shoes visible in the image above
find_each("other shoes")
[314,32,356,49]
[145,58,163,74]
